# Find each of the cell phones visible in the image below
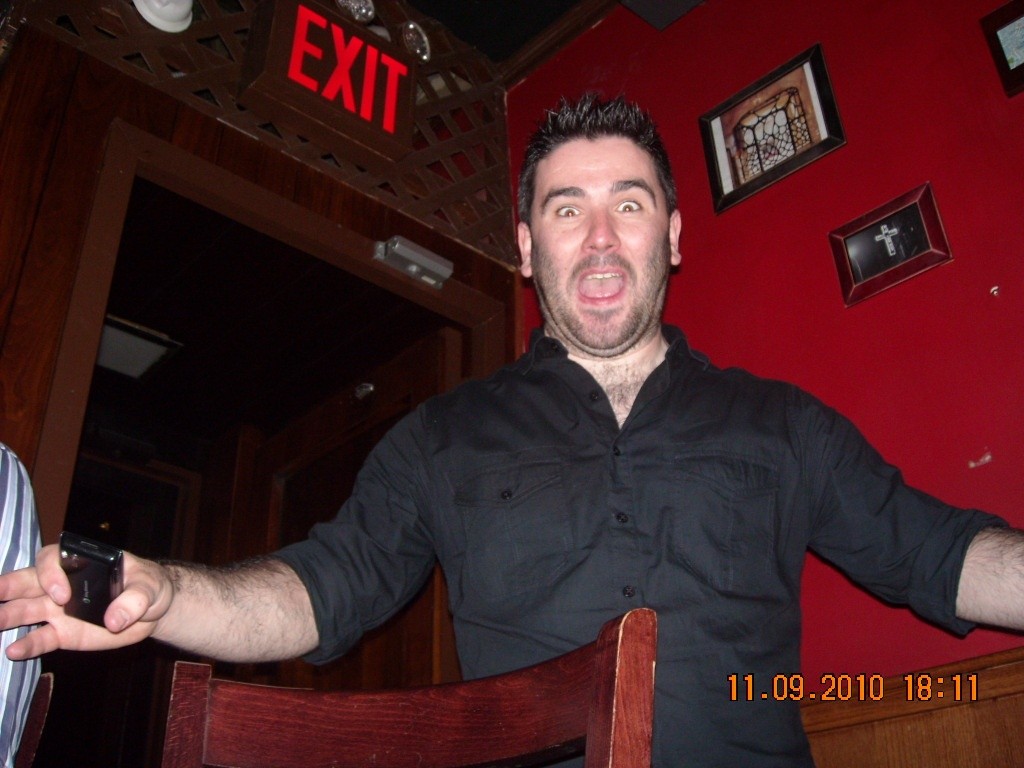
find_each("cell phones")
[58,532,124,629]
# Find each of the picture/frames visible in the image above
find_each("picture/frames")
[979,0,1024,96]
[699,42,847,215]
[830,182,953,307]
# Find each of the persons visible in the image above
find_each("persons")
[1,89,1023,768]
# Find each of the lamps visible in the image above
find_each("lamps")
[337,0,376,24]
[399,20,431,66]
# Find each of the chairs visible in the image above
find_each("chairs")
[159,607,657,768]
